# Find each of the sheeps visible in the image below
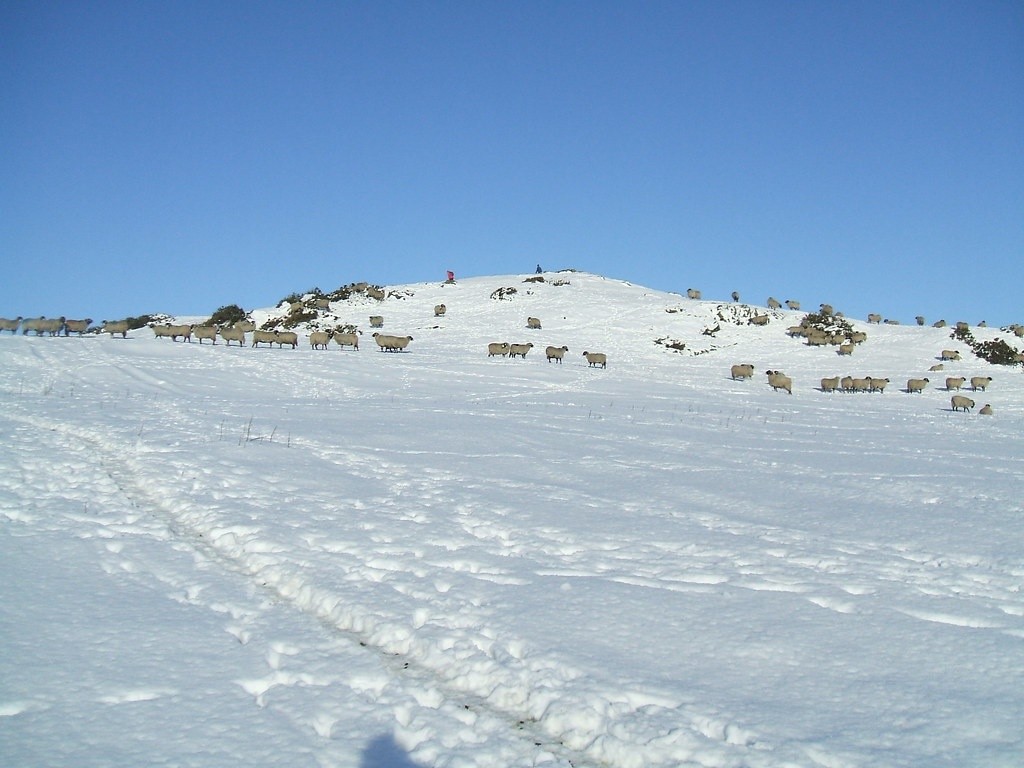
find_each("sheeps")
[839,340,854,355]
[65,319,93,337]
[934,320,946,328]
[235,320,256,332]
[915,317,925,326]
[731,364,754,380]
[980,404,993,415]
[350,282,367,295]
[832,335,846,345]
[1011,352,1024,364]
[332,331,359,351]
[546,346,568,364]
[687,289,700,299]
[819,304,833,315]
[101,320,129,338]
[372,332,413,353]
[488,342,510,358]
[434,304,446,316]
[884,319,899,325]
[841,376,853,390]
[0,317,22,335]
[870,378,890,394]
[251,330,298,350]
[821,377,840,392]
[732,292,739,302]
[850,331,866,345]
[190,324,217,345]
[165,323,191,343]
[774,371,785,376]
[785,300,800,310]
[956,322,969,330]
[367,287,384,298]
[825,335,832,344]
[310,332,333,350]
[942,350,959,362]
[954,355,962,360]
[946,377,966,390]
[951,396,975,413]
[527,317,541,330]
[290,301,304,311]
[369,316,384,326]
[149,323,169,339]
[809,335,826,346]
[978,321,986,327]
[766,370,792,394]
[971,377,993,392]
[314,298,329,307]
[768,301,782,310]
[1011,324,1018,331]
[907,378,930,394]
[1015,326,1024,338]
[220,326,245,347]
[930,364,944,371]
[803,327,816,337]
[583,351,606,369]
[509,343,534,359]
[812,330,828,338]
[835,312,844,316]
[748,316,768,325]
[868,314,881,325]
[786,327,803,337]
[22,316,65,337]
[852,376,871,393]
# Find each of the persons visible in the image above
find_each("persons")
[536,263,543,274]
[447,270,455,280]
